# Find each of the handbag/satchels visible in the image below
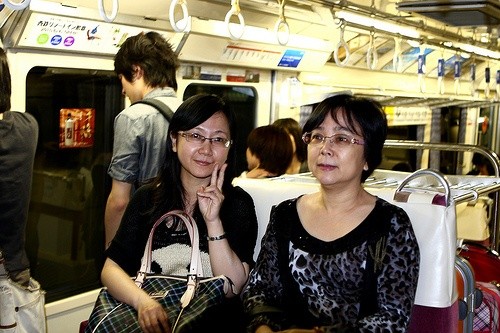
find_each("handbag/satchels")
[85,210,236,333]
[473,282,500,333]
[0,249,46,333]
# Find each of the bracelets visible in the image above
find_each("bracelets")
[207,232,226,240]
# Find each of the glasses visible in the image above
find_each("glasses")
[301,132,366,147]
[177,131,233,148]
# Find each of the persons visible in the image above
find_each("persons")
[100,94,258,333]
[468,151,496,175]
[105,32,185,254]
[241,94,420,333]
[232,125,293,179]
[0,47,38,287]
[272,118,307,174]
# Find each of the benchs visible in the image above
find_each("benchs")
[230,176,461,333]
